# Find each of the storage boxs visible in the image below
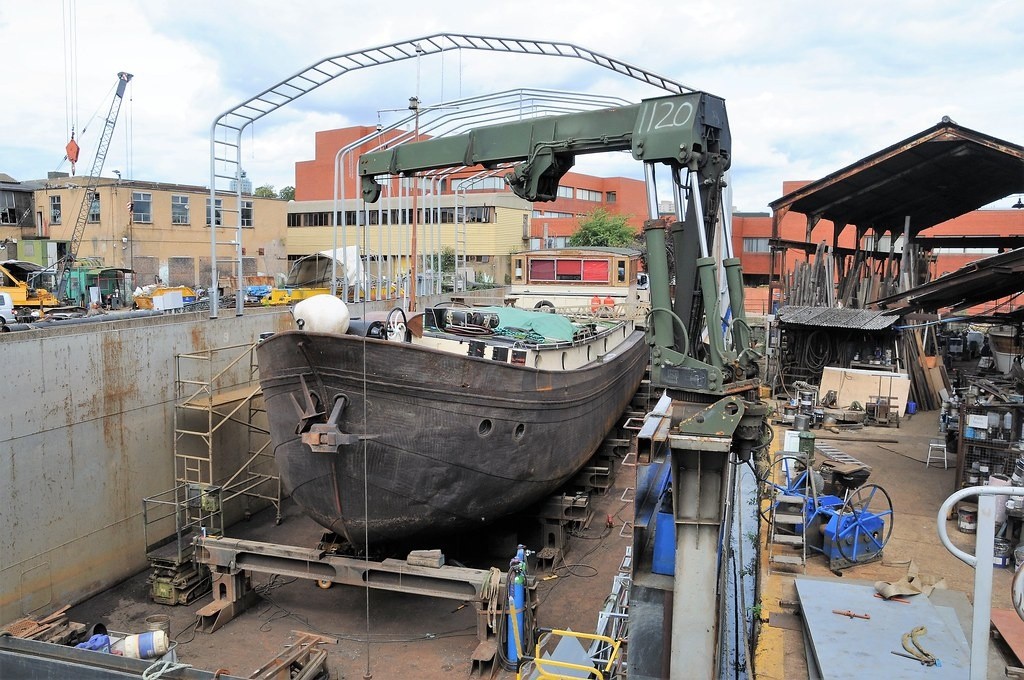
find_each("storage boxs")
[152,290,183,311]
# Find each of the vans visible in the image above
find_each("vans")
[0,292,18,327]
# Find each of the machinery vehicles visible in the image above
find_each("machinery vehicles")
[0,70,133,322]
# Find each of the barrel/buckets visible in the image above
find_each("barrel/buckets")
[125,630,169,659]
[957,506,977,534]
[1014,542,1024,571]
[145,614,170,638]
[966,468,980,486]
[993,538,1011,568]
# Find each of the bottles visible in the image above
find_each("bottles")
[1004,412,1012,429]
[604,295,614,309]
[592,294,601,312]
[908,401,916,414]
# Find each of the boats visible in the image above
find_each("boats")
[261,244,403,305]
[502,244,651,331]
[255,292,654,563]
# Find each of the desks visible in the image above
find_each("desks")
[849,360,897,374]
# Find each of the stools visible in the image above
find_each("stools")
[926,438,948,471]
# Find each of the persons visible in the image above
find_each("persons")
[590,294,601,312]
[602,295,615,311]
